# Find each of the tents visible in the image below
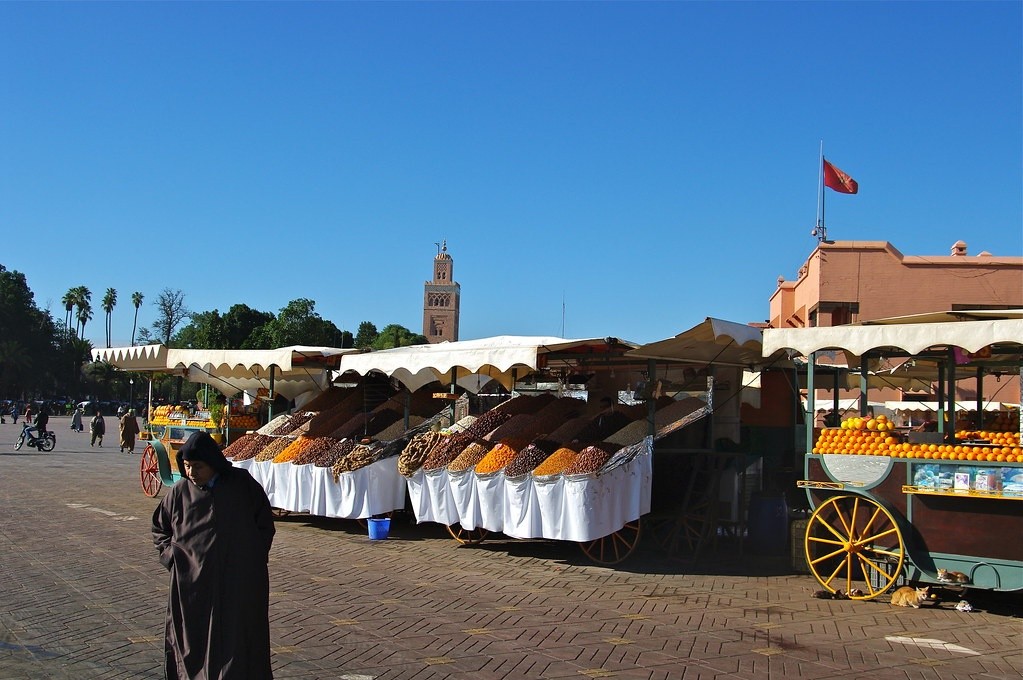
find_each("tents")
[91,317,1023,441]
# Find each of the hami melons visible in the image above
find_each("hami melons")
[153,405,185,416]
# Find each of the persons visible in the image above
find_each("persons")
[71,409,82,433]
[119,409,140,453]
[25,402,31,423]
[25,407,48,451]
[117,404,129,420]
[215,395,226,406]
[0,403,8,416]
[599,397,614,411]
[90,411,105,448]
[142,404,148,428]
[151,431,276,680]
[11,404,18,424]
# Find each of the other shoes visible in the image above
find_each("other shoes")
[38,448,41,451]
[128,451,133,454]
[89,443,94,448]
[28,438,32,443]
[98,445,102,448]
[120,447,124,453]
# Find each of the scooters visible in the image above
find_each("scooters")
[14,421,56,451]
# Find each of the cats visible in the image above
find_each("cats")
[890,585,930,608]
[936,567,970,584]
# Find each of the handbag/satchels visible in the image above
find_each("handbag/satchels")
[80,424,83,431]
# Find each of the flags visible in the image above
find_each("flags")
[824,159,858,194]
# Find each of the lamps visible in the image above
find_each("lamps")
[626,383,631,394]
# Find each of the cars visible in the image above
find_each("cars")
[0,399,129,415]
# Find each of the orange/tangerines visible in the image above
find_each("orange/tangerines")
[813,411,1023,463]
[149,416,217,428]
[227,416,259,429]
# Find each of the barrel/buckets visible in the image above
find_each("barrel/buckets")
[210,433,222,444]
[367,518,391,539]
